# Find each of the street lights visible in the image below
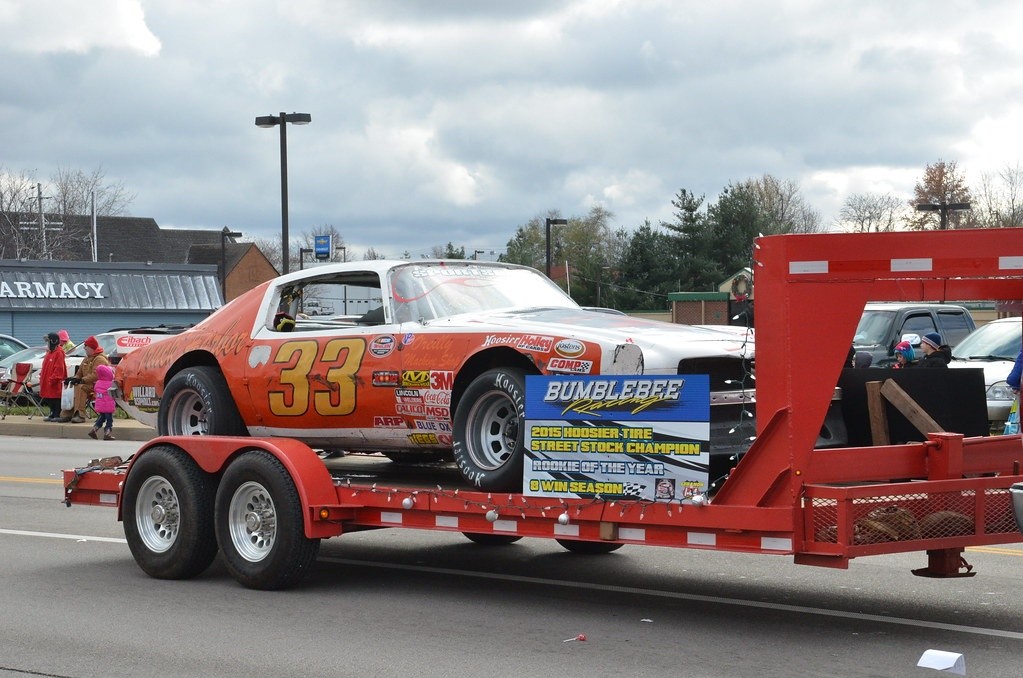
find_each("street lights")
[917,204,972,229]
[255,111,311,276]
[474,250,484,261]
[546,218,567,277]
[221,231,242,304]
[299,248,313,270]
[337,246,346,262]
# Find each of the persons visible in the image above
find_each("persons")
[57,330,73,352]
[58,337,109,423]
[284,290,302,305]
[1006,352,1023,433]
[892,340,919,368]
[39,332,67,422]
[88,365,118,440]
[918,332,951,368]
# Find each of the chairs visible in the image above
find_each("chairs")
[0,363,99,422]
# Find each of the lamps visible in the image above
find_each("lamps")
[147,261,152,266]
[21,258,27,262]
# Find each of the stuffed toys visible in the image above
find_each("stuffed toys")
[273,313,295,332]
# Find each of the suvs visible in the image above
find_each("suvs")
[63,326,200,403]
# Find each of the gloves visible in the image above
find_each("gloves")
[71,378,81,386]
[61,377,74,386]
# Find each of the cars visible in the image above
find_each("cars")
[945,317,1022,421]
[0,345,48,394]
[851,302,976,366]
[1,334,30,362]
[113,258,759,492]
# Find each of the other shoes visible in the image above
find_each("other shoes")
[51,417,59,422]
[103,433,115,440]
[71,415,85,423]
[88,429,99,440]
[58,416,73,423]
[43,416,53,421]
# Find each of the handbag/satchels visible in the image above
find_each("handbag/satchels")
[61,380,74,410]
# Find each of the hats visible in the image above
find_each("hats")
[84,336,98,350]
[57,330,69,342]
[922,332,942,350]
[894,341,915,363]
[48,332,60,353]
